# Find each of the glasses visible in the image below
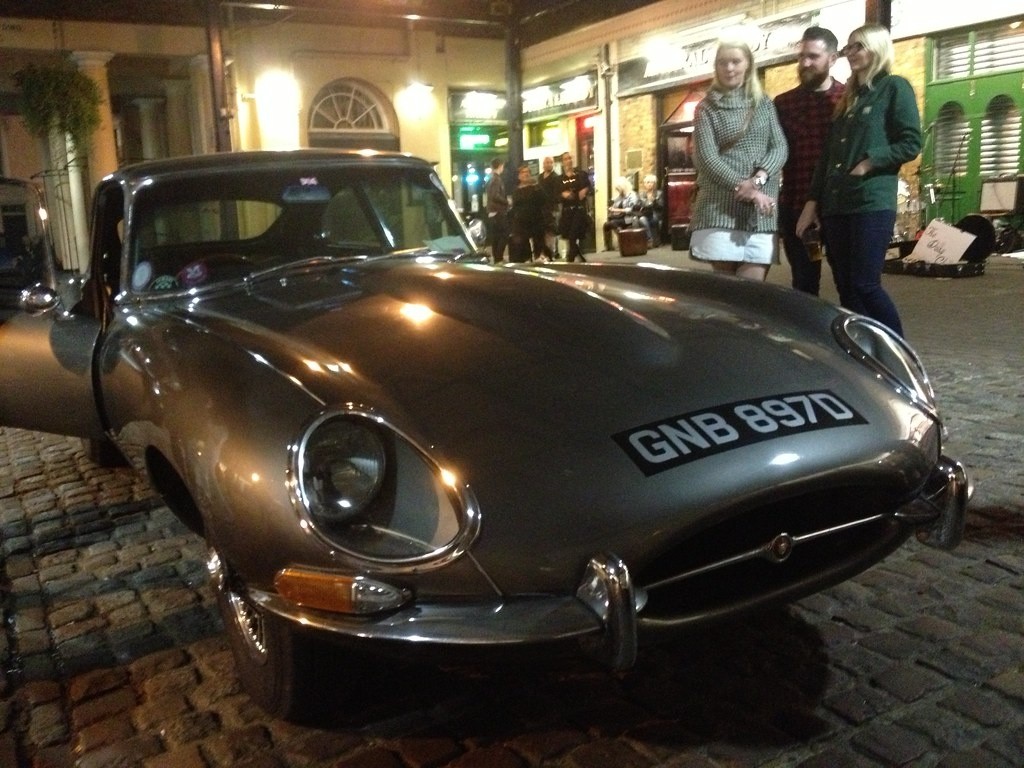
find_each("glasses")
[842,42,867,56]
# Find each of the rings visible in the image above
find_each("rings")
[771,202,775,206]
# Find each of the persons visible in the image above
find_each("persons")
[686,23,921,339]
[486,153,591,263]
[601,174,665,251]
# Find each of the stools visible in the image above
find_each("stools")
[619,228,647,256]
[672,223,692,251]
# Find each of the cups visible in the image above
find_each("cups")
[801,229,823,262]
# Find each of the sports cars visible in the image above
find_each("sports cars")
[0,151,971,722]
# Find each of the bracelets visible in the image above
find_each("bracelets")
[751,174,766,185]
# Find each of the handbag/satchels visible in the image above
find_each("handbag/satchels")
[687,180,701,206]
